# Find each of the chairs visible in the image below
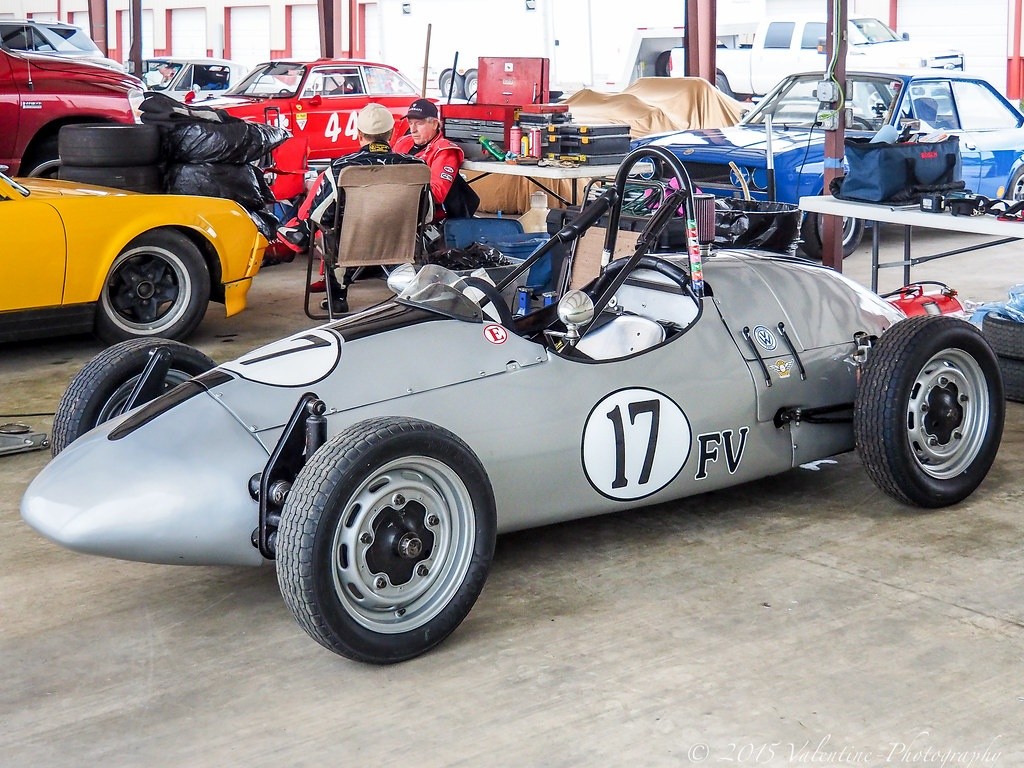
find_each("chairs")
[305,163,431,322]
[559,313,666,360]
[907,97,939,130]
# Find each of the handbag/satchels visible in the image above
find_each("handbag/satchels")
[842,135,963,200]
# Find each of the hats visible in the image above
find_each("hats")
[357,103,395,134]
[399,99,438,121]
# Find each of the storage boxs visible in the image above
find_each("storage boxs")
[440,103,631,165]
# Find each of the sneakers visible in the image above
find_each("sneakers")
[277,220,314,252]
[311,279,327,292]
[321,297,348,312]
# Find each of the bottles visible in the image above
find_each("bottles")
[478,136,506,161]
[520,133,530,157]
[510,121,521,155]
[528,124,542,159]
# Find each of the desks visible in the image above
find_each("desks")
[798,194,1024,302]
[458,156,654,207]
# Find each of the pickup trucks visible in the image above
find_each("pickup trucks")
[669,12,964,100]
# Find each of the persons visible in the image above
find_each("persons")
[309,100,465,292]
[273,103,426,313]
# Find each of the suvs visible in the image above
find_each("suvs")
[0,18,105,58]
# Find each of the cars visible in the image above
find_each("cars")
[0,35,152,180]
[629,68,1024,263]
[141,57,250,105]
[186,57,474,162]
[0,163,270,349]
